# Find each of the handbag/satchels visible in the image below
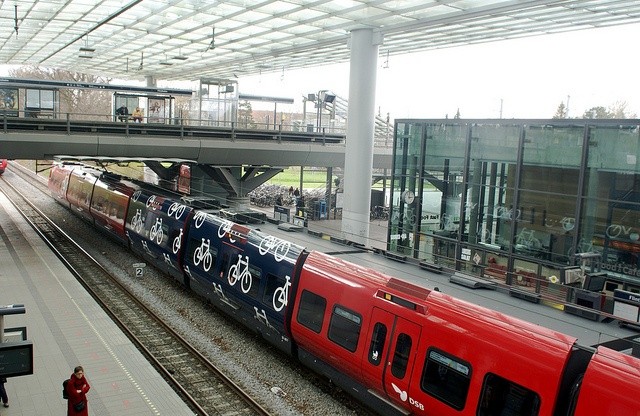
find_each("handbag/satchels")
[73,393,84,412]
[118,113,122,119]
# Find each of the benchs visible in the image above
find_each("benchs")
[119,112,139,123]
[28,108,53,119]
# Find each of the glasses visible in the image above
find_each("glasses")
[75,373,83,375]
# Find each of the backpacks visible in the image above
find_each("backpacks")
[63,379,68,399]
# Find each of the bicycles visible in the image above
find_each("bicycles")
[150,217,163,245]
[132,191,142,202]
[168,202,194,219]
[495,207,521,221]
[184,266,200,285]
[606,224,640,242]
[259,236,291,262]
[568,239,599,264]
[212,282,239,311]
[141,240,155,260]
[478,223,490,242]
[272,275,291,312]
[163,253,179,272]
[511,228,541,254]
[250,184,325,216]
[227,254,252,293]
[193,238,213,272]
[146,194,160,211]
[193,210,207,228]
[173,228,184,254]
[218,220,242,243]
[545,216,576,231]
[370,206,388,221]
[392,208,417,226]
[131,209,142,233]
[253,307,280,335]
[434,213,456,232]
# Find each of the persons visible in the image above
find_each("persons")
[116,102,128,121]
[132,106,142,122]
[289,185,293,193]
[67,365,90,416]
[294,187,299,195]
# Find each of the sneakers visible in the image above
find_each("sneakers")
[4,402,9,407]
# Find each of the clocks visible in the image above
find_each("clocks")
[402,191,415,204]
